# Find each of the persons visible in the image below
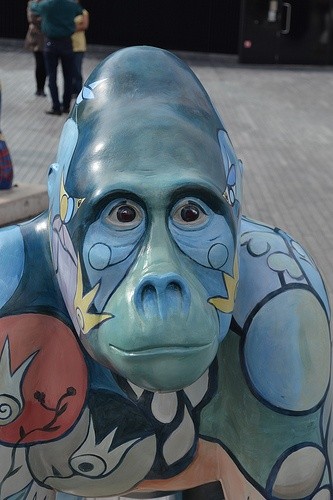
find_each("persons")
[24,0,88,115]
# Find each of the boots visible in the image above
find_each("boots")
[44,84,61,115]
[59,85,71,113]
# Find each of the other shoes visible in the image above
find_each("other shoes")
[36,89,45,96]
